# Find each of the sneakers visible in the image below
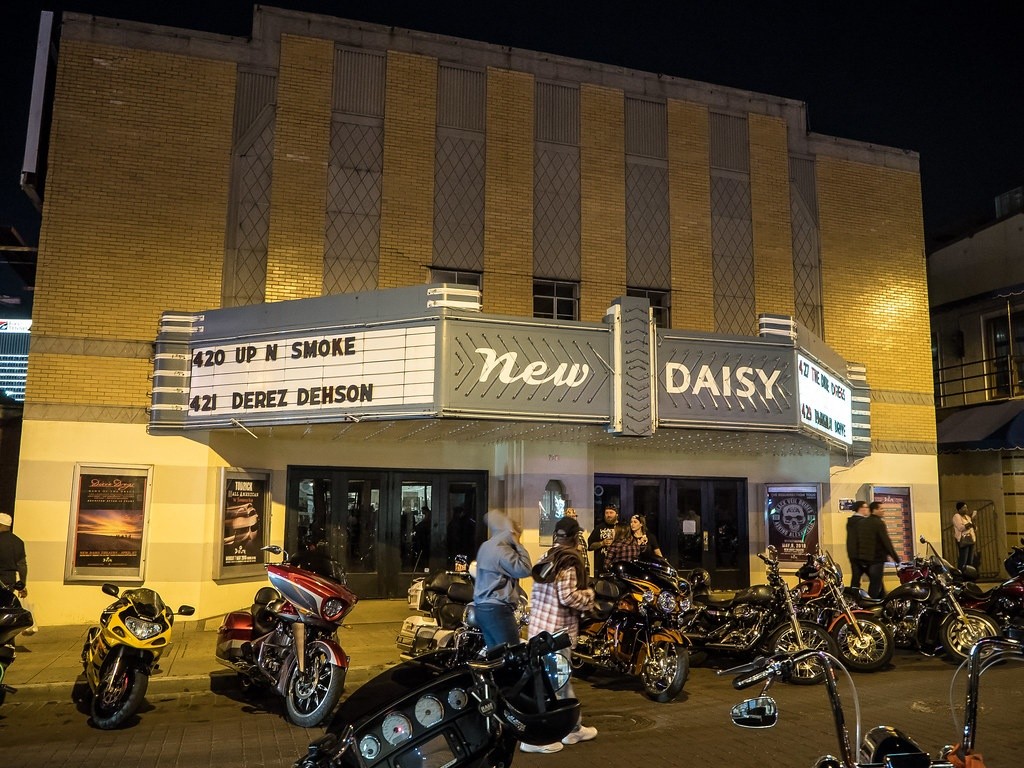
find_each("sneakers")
[520,739,563,754]
[562,726,597,745]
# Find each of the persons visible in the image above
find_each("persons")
[0,512,28,600]
[953,501,978,572]
[861,501,902,601]
[518,516,598,753]
[562,503,663,588]
[472,509,533,650]
[846,501,871,588]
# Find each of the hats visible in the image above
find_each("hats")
[0,513,12,526]
[554,516,585,537]
[606,503,618,514]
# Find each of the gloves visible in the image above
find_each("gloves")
[18,587,28,599]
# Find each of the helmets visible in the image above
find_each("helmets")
[502,693,580,745]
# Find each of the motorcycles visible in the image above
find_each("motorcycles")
[291,631,581,768]
[841,535,1024,664]
[0,580,33,706]
[571,560,712,703]
[71,584,195,730]
[395,551,531,674]
[630,543,895,686]
[716,635,1024,768]
[209,539,373,728]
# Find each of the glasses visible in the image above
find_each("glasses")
[234,522,259,535]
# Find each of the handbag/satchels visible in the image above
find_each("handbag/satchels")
[19,597,38,637]
